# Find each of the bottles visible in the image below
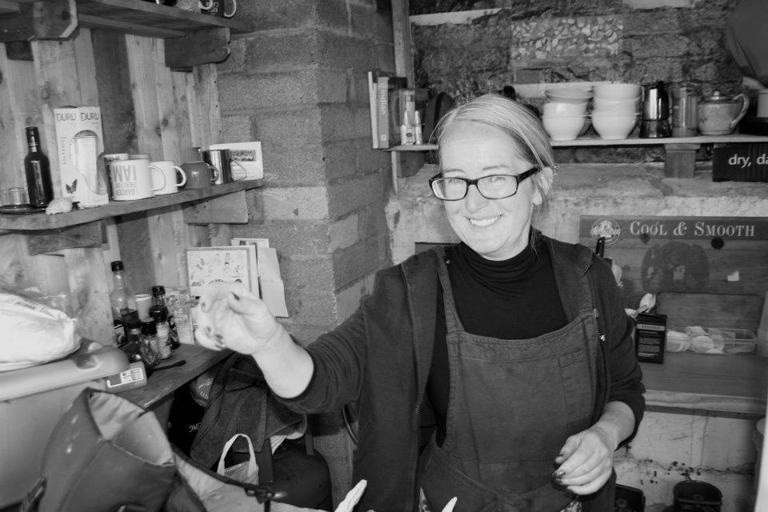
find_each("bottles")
[107,260,179,377]
[23,126,53,208]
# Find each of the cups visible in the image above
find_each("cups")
[152,161,186,195]
[203,148,246,186]
[8,187,24,206]
[669,81,702,138]
[639,81,673,138]
[179,161,219,188]
[150,1,239,20]
[101,152,166,201]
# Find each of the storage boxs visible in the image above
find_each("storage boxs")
[0,338,129,509]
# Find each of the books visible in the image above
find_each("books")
[367,69,430,151]
[186,240,266,333]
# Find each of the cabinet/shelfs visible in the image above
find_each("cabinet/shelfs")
[0,0,264,412]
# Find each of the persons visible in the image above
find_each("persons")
[193,92,650,511]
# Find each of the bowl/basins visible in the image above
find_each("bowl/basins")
[542,75,644,144]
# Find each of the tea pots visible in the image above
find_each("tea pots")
[696,89,748,135]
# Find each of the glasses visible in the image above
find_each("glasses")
[429,167,539,201]
